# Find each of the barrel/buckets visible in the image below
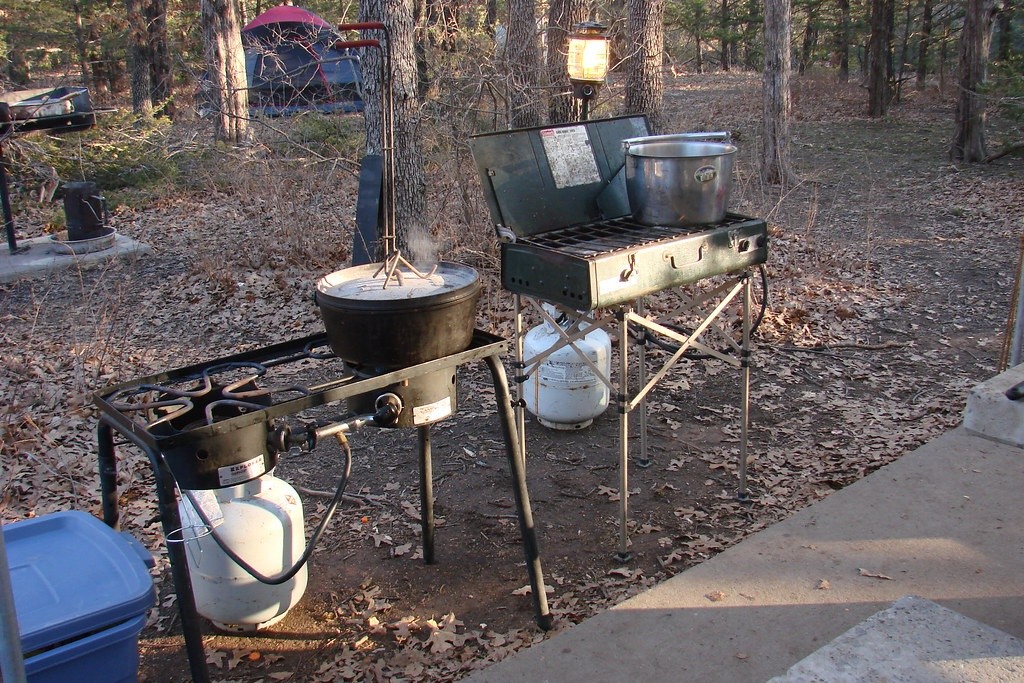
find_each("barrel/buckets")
[62,179,106,243]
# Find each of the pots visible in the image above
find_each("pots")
[317,249,481,375]
[623,131,737,227]
[9,93,75,118]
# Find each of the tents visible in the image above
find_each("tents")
[185,6,365,117]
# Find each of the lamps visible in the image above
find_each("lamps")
[566,21,612,122]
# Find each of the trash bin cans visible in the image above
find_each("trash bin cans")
[3,511,156,683]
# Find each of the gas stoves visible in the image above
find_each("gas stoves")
[93,311,521,489]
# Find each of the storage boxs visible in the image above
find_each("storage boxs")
[0,512,156,683]
